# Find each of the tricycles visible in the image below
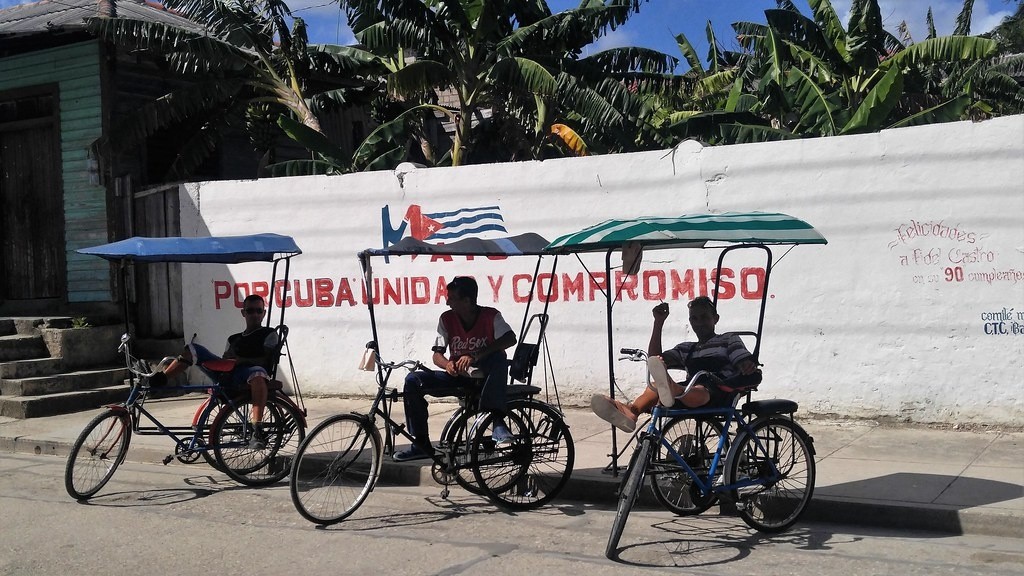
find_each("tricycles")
[289,231,577,525]
[541,211,818,561]
[64,233,308,502]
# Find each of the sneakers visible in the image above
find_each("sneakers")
[137,359,167,397]
[492,422,516,443]
[394,443,434,461]
[249,426,265,450]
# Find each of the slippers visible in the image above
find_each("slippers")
[591,394,637,433]
[648,355,676,408]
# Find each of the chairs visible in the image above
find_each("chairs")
[659,332,759,418]
[460,314,549,409]
[242,326,289,393]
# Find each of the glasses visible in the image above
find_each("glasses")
[243,307,264,315]
[688,298,715,313]
[453,277,464,297]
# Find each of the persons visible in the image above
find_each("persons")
[135,295,279,450]
[393,276,517,462]
[591,295,758,433]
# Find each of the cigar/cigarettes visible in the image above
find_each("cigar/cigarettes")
[658,297,664,303]
